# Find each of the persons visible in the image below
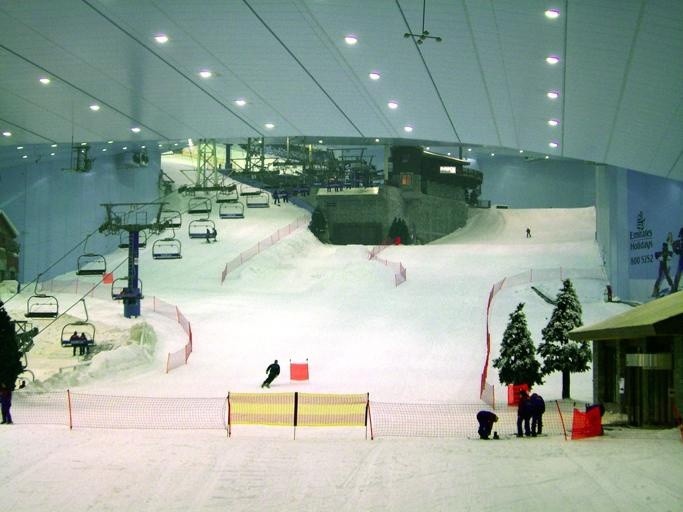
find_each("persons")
[18,381,25,389]
[79,332,88,354]
[260,359,280,388]
[514,390,531,436]
[281,191,288,203]
[0,380,13,424]
[525,227,531,238]
[68,330,82,356]
[530,393,544,436]
[205,229,210,243]
[212,227,217,242]
[272,191,280,204]
[476,410,499,439]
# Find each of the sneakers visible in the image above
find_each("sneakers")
[0,421,13,424]
[480,431,542,440]
